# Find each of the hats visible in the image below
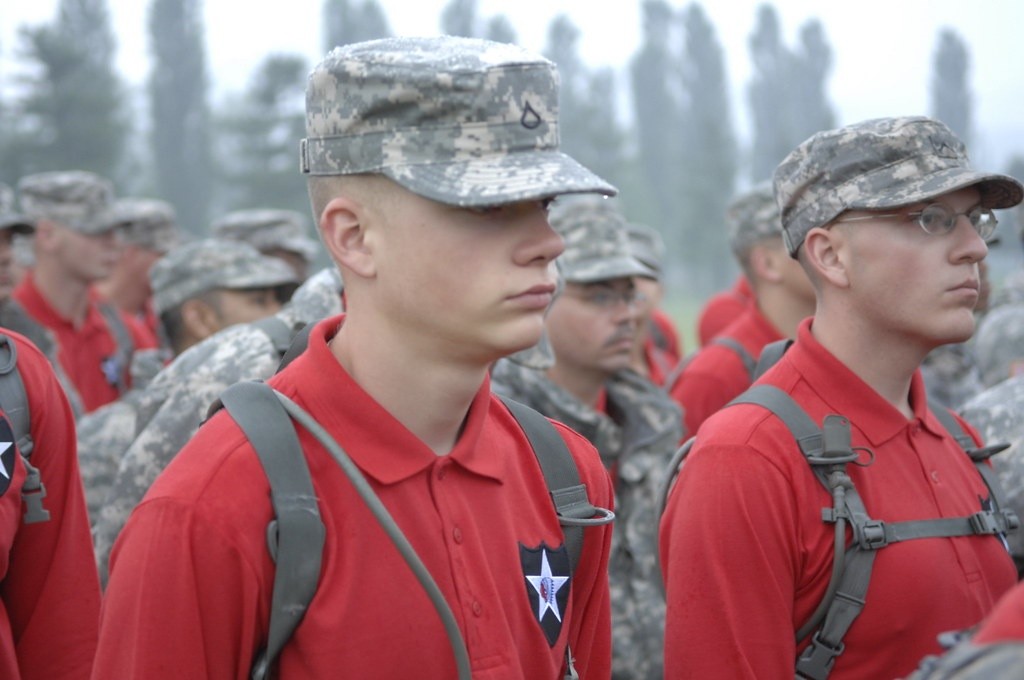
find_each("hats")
[0,183,35,236]
[147,238,300,320]
[627,224,665,280]
[299,34,622,209]
[724,181,782,251]
[212,208,318,263]
[542,204,658,283]
[772,115,1024,258]
[20,170,133,236]
[116,201,186,253]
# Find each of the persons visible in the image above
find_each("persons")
[92,33,615,680]
[0,117,1024,680]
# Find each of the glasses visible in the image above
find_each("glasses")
[827,202,999,240]
[556,283,649,316]
